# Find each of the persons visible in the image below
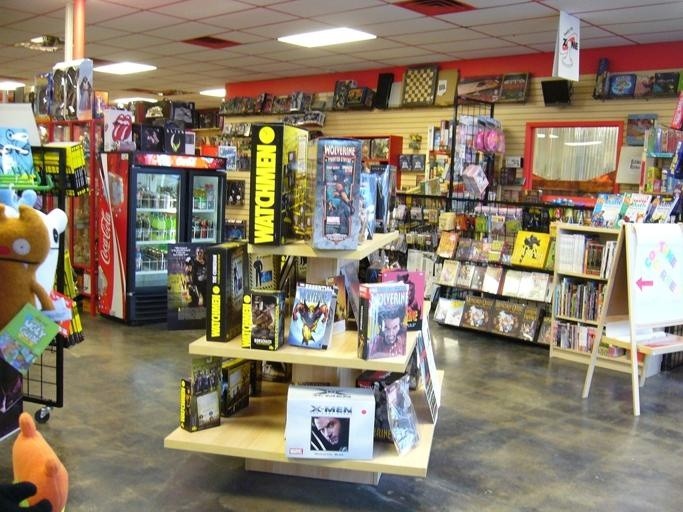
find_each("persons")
[368,305,406,358]
[313,417,349,453]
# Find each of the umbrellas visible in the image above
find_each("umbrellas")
[295,255,306,283]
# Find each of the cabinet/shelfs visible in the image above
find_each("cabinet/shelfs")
[162,233,445,485]
[37,118,103,319]
[337,134,404,190]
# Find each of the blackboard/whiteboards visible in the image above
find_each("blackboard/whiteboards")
[626,224,683,328]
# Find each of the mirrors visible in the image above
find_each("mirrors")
[522,119,626,196]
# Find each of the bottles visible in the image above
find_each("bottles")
[137,213,175,241]
[191,217,212,238]
[191,185,214,209]
[136,184,174,209]
[136,245,168,270]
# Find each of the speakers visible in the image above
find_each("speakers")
[373,72,392,108]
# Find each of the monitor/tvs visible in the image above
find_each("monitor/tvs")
[541,78,570,106]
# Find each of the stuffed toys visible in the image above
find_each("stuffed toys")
[4,205,67,313]
[12,411,68,512]
[0,203,54,332]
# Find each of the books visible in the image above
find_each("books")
[554,277,608,323]
[640,126,682,156]
[557,232,617,280]
[554,321,625,357]
[432,290,552,348]
[590,193,625,227]
[618,191,652,228]
[437,259,552,303]
[644,156,682,193]
[0,302,60,377]
[643,194,681,224]
[436,231,556,272]
